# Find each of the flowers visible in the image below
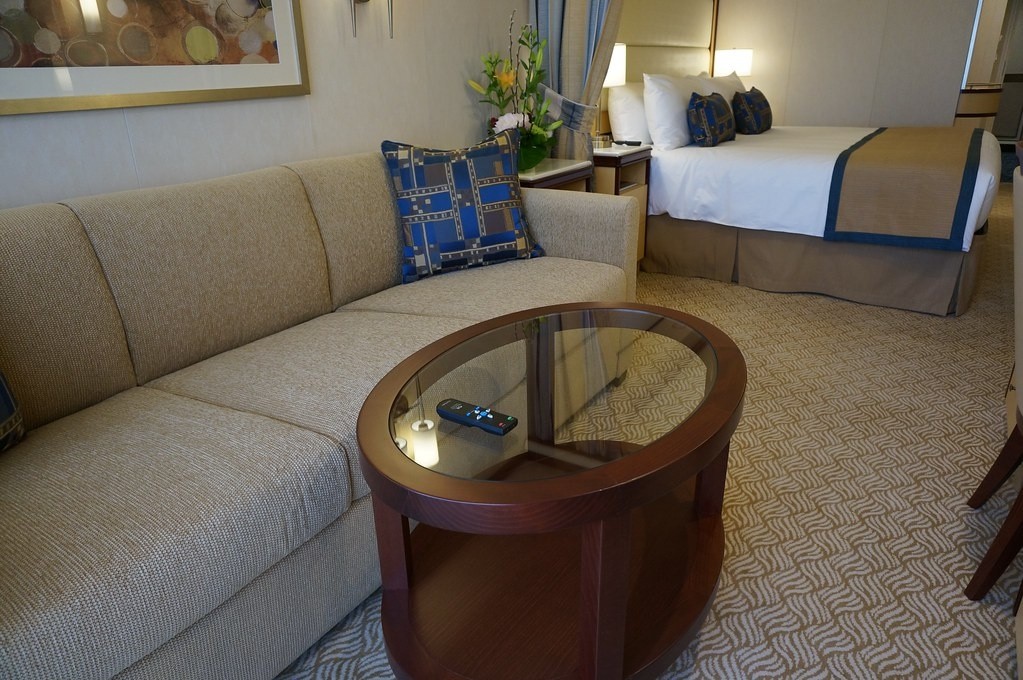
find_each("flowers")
[468,9,563,147]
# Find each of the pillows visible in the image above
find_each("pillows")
[381,126,546,286]
[608,81,652,145]
[705,70,747,115]
[732,85,772,135]
[697,71,710,81]
[642,72,716,151]
[687,91,735,147]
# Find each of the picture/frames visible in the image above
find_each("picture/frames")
[0,0,311,116]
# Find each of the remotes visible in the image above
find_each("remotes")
[435,399,518,436]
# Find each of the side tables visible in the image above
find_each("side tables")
[517,158,593,192]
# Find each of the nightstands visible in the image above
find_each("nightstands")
[591,144,653,261]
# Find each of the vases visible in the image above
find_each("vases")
[488,132,548,170]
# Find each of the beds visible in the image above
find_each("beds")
[602,70,1001,317]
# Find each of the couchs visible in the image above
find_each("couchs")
[0,126,639,680]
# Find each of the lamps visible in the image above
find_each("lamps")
[713,47,752,77]
[589,43,626,148]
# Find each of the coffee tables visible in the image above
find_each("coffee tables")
[355,301,748,680]
